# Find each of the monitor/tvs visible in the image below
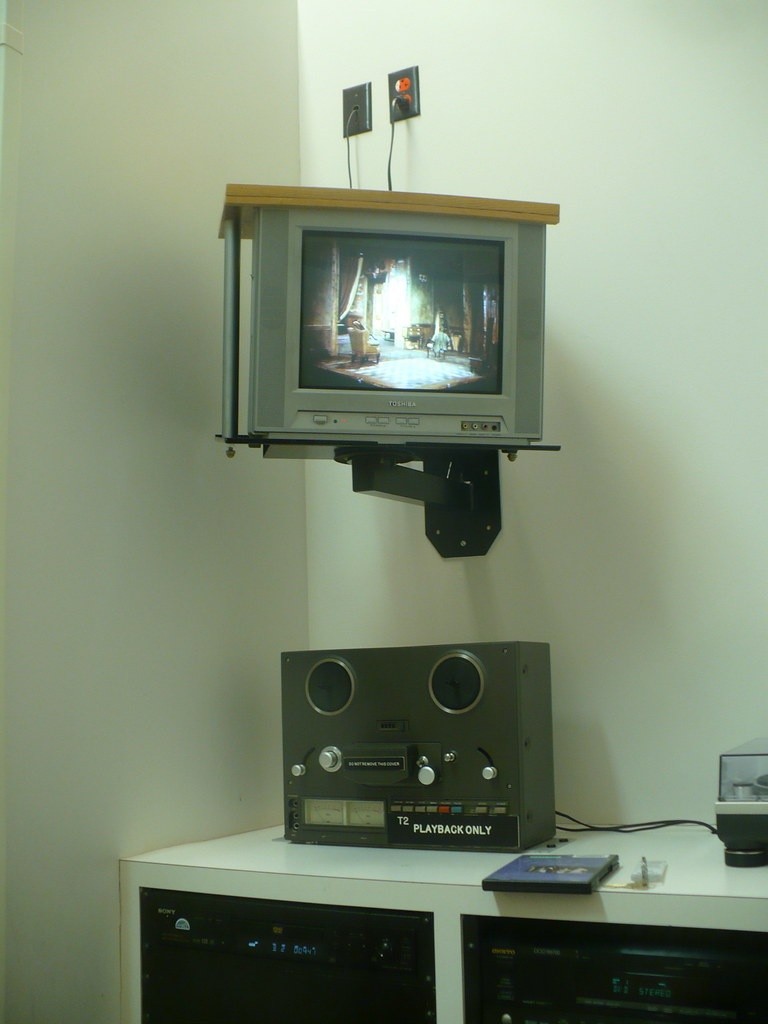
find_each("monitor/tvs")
[247,204,546,443]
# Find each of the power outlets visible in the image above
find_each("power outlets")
[388,65,420,123]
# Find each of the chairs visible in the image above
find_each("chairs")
[349,328,380,364]
[405,327,422,349]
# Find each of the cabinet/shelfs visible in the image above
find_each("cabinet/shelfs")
[123,822,768,1024]
